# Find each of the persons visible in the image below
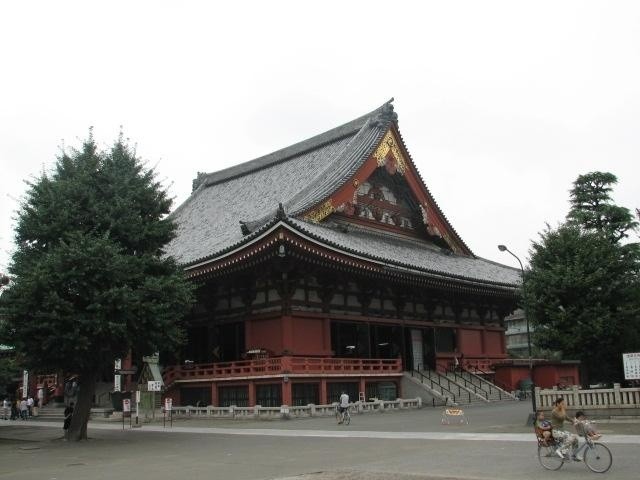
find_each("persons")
[573,409,601,441]
[61,402,75,442]
[550,397,582,463]
[533,409,553,447]
[2,393,39,421]
[337,389,350,425]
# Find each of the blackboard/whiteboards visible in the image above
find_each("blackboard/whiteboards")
[622,353,639,379]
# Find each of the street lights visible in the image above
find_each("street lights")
[498,244,535,412]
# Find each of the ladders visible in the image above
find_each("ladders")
[359,392,365,401]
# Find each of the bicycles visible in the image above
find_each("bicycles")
[538,420,612,473]
[334,404,351,425]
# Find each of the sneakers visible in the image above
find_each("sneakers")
[573,456,582,462]
[555,449,564,459]
